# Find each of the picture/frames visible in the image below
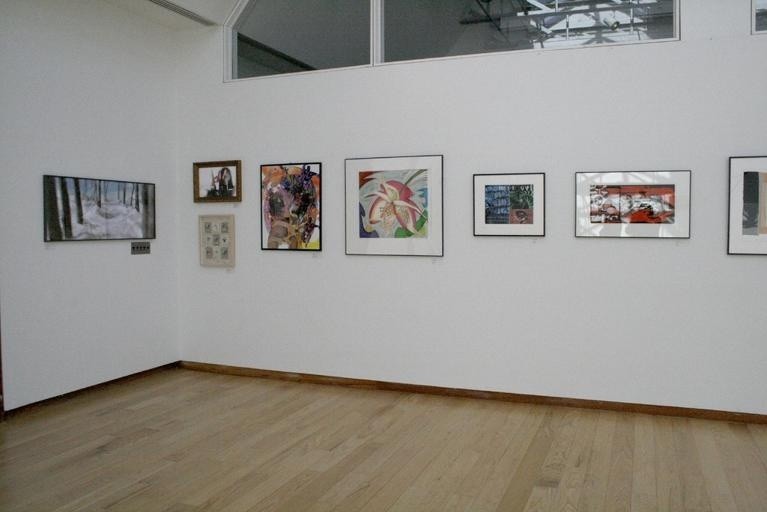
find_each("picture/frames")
[261,162,321,251]
[473,173,545,236]
[44,175,155,241]
[345,155,443,256]
[575,170,690,238]
[193,160,242,202]
[728,156,767,255]
[198,214,235,267]
